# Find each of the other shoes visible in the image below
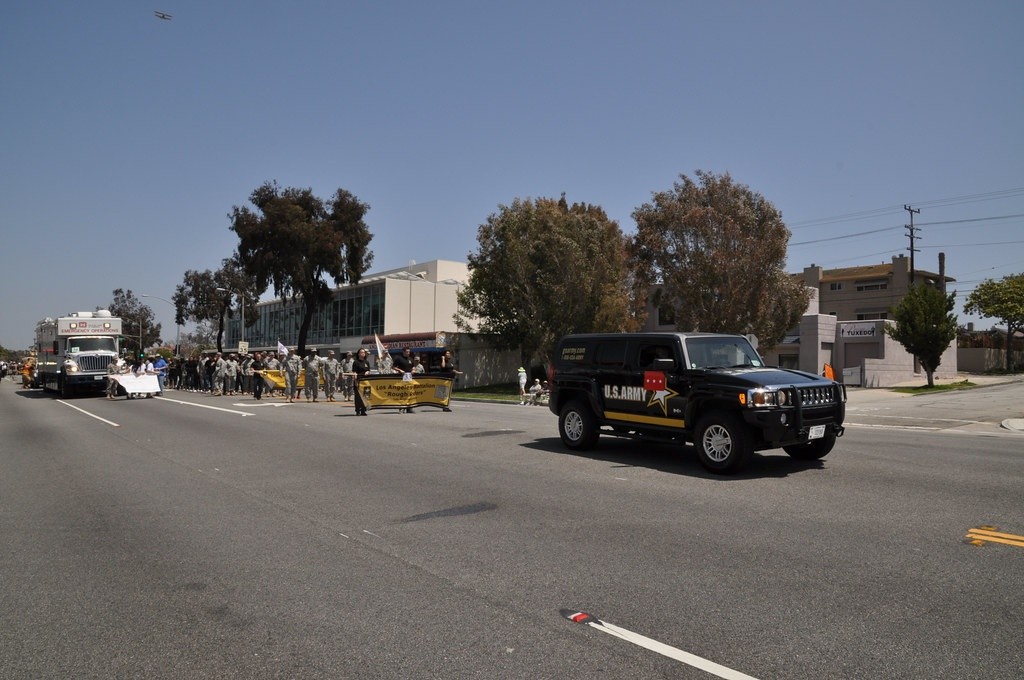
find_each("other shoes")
[306,397,311,403]
[313,398,319,403]
[106,395,115,399]
[327,397,336,402]
[520,401,541,406]
[355,410,367,416]
[443,408,452,412]
[344,395,353,401]
[399,408,415,414]
[163,386,285,400]
[286,395,295,403]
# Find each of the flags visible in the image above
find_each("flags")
[276,341,289,356]
[373,334,392,358]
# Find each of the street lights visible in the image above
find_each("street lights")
[142,294,179,354]
[217,288,245,341]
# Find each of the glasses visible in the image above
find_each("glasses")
[544,383,547,384]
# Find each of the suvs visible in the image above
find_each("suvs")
[548,332,848,476]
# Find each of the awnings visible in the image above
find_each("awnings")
[360,331,446,353]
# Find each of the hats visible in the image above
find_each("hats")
[311,348,317,351]
[269,352,274,355]
[289,347,294,351]
[518,367,525,371]
[383,349,388,353]
[327,350,335,355]
[113,356,118,360]
[414,357,419,360]
[346,351,352,356]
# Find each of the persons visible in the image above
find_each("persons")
[0,358,31,389]
[105,354,168,399]
[166,347,463,416]
[518,367,550,407]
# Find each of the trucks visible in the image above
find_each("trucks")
[33,310,123,398]
